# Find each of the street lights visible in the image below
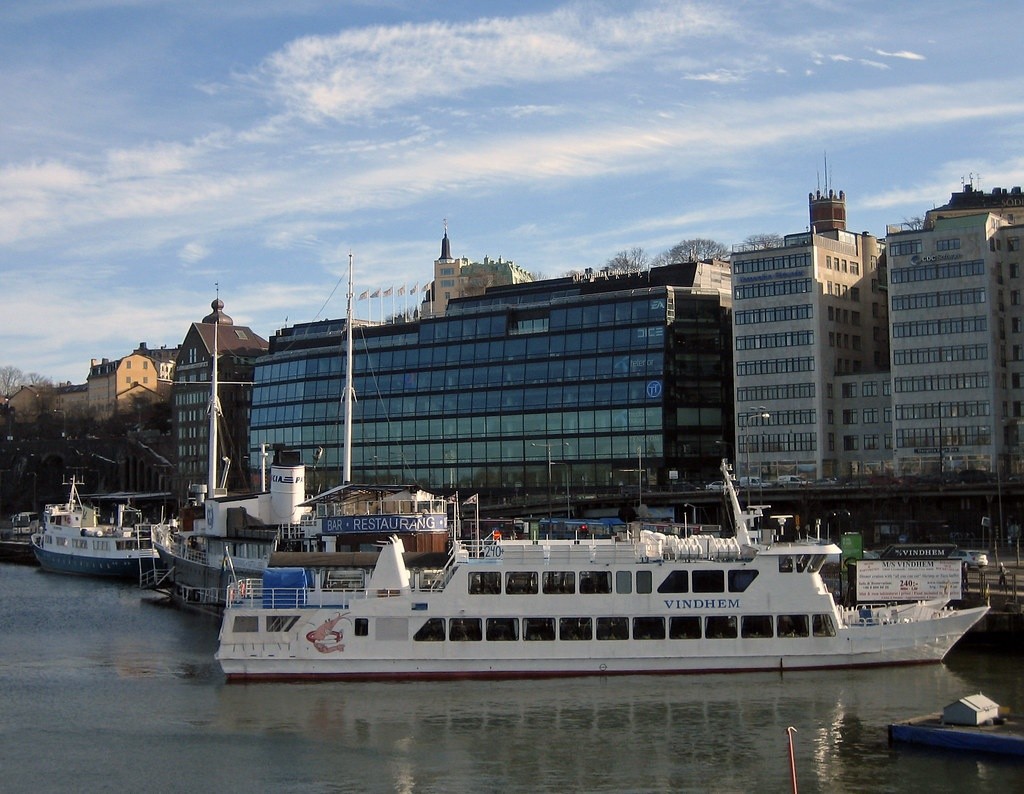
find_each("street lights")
[750,406,765,505]
[715,440,736,473]
[683,501,696,522]
[549,462,571,520]
[745,413,770,530]
[530,442,570,541]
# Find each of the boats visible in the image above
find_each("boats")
[0,249,997,682]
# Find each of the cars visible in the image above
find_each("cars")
[667,482,700,491]
[777,474,813,487]
[957,549,989,568]
[739,476,773,488]
[705,479,727,490]
[815,475,842,486]
[622,484,652,496]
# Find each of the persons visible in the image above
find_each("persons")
[962,561,970,592]
[999,561,1010,595]
